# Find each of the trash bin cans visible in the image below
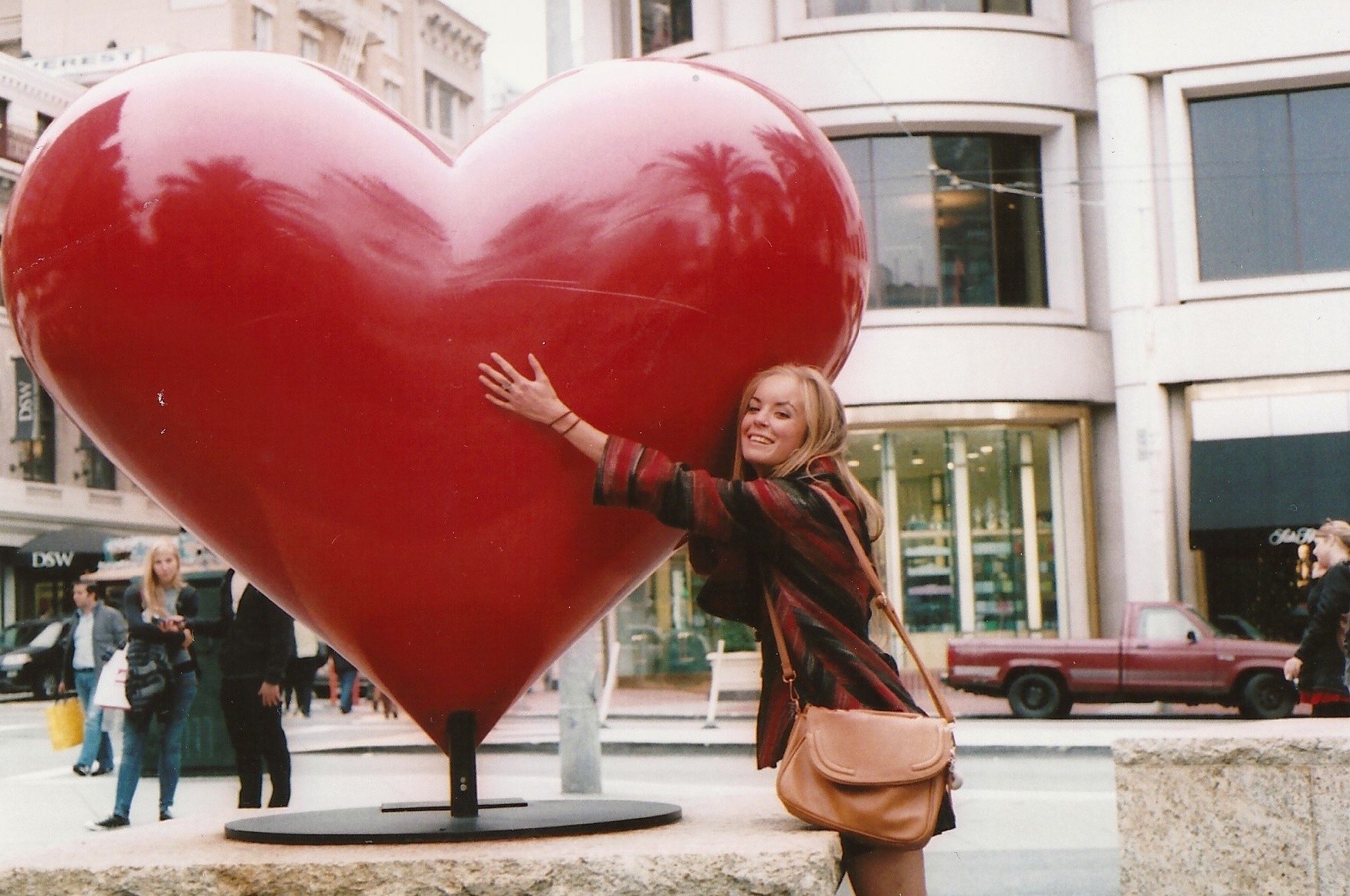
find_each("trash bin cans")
[86,569,236,776]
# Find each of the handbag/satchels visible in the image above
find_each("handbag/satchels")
[46,690,84,751]
[776,703,952,851]
[126,659,176,707]
[314,642,329,668]
[93,643,131,711]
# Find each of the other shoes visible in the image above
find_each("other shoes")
[160,810,173,820]
[95,813,130,827]
[91,768,113,775]
[73,765,89,776]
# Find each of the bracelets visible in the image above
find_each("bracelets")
[547,409,573,429]
[561,415,583,436]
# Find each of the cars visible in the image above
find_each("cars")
[0,609,77,701]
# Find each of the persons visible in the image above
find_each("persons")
[283,619,400,724]
[55,577,128,779]
[158,568,294,809]
[85,541,199,831]
[477,350,955,896]
[1283,518,1350,717]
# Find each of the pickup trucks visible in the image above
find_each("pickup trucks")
[939,596,1312,721]
